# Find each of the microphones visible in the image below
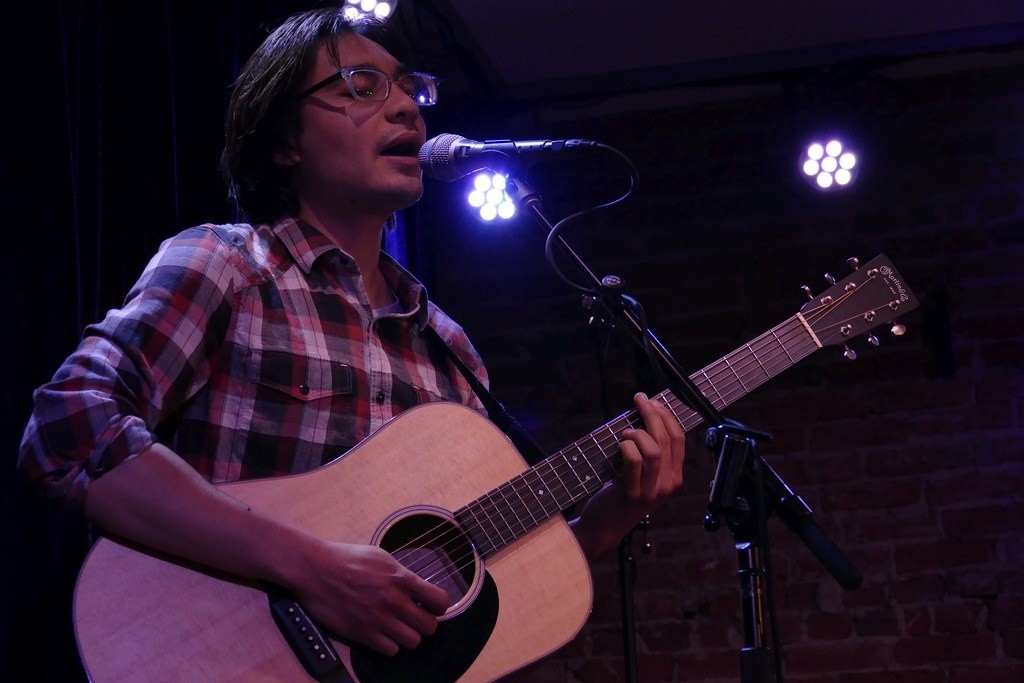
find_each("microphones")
[419,133,595,183]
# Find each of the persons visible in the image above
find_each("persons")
[17,10,688,683]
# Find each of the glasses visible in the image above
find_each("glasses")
[287,66,442,108]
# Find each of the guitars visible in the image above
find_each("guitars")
[71,251,924,682]
